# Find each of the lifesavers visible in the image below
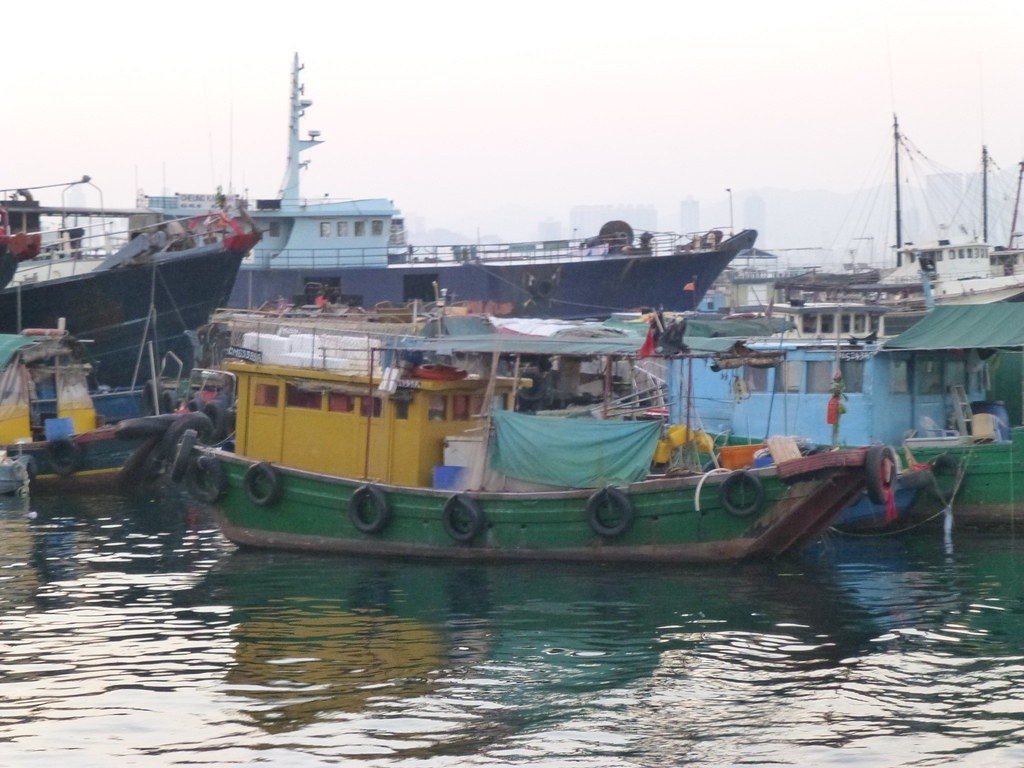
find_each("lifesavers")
[441,494,481,542]
[516,366,547,404]
[242,462,280,506]
[718,469,764,516]
[416,362,468,382]
[348,483,391,534]
[46,435,81,476]
[921,452,964,500]
[534,276,557,301]
[586,487,635,539]
[112,378,237,503]
[863,444,898,506]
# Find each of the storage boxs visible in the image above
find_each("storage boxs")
[430,466,467,492]
[442,436,488,467]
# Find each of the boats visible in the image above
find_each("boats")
[2,50,1024,535]
[112,287,903,566]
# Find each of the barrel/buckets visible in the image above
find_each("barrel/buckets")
[56,396,95,434]
[970,400,1010,441]
[432,465,473,491]
[45,417,75,441]
[718,443,775,468]
[0,403,32,445]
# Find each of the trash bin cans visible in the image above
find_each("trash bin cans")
[966,401,1009,438]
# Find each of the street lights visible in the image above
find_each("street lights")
[727,189,734,236]
